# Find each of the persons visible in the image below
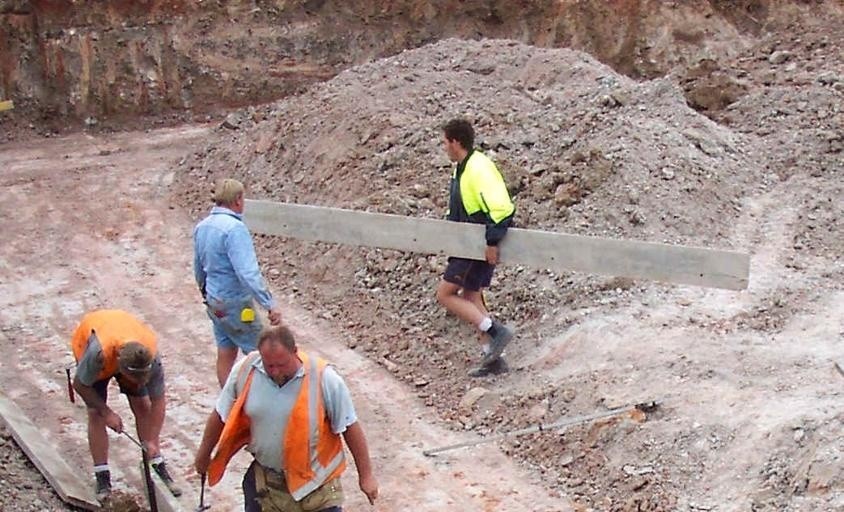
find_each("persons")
[191,177,285,393]
[69,306,184,503]
[191,321,380,512]
[435,118,517,380]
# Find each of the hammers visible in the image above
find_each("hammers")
[193,472,213,512]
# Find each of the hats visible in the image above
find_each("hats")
[118,341,155,376]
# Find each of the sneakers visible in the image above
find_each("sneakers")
[151,459,183,498]
[467,353,509,379]
[95,469,113,501]
[482,321,513,366]
